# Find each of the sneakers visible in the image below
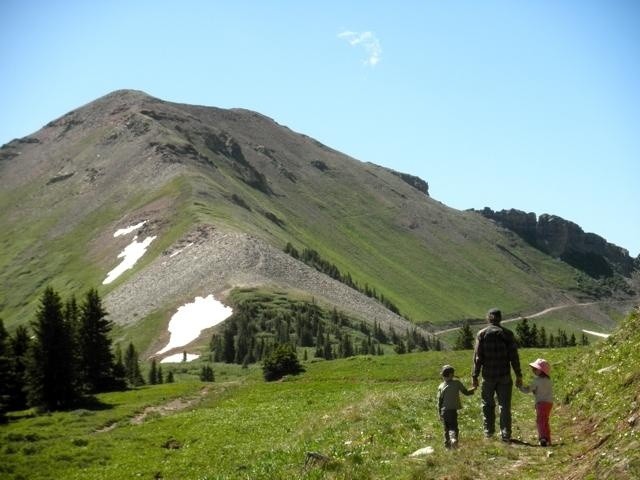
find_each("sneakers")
[541,438,553,447]
[443,438,460,447]
[483,430,513,442]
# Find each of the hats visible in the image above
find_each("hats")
[439,364,455,377]
[488,308,501,318]
[528,358,551,377]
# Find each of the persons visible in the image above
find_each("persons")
[519,359,554,445]
[471,308,523,442]
[436,365,477,451]
